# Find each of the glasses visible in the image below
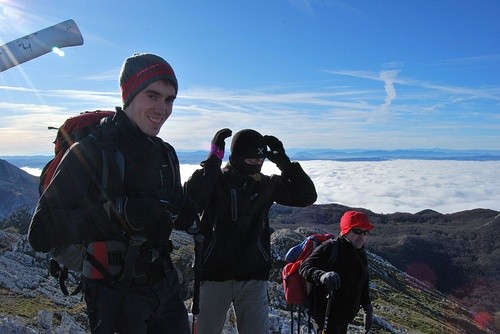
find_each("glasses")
[351,228,371,237]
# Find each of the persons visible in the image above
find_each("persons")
[193,129,318,334]
[298,210,374,334]
[27,53,233,334]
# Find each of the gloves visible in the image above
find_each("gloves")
[127,196,185,230]
[175,198,197,231]
[263,135,291,171]
[210,128,232,158]
[320,271,341,292]
[364,313,373,332]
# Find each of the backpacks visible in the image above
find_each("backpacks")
[282,232,339,305]
[38,111,180,273]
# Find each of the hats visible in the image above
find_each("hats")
[229,129,268,176]
[121,54,179,109]
[340,211,375,236]
